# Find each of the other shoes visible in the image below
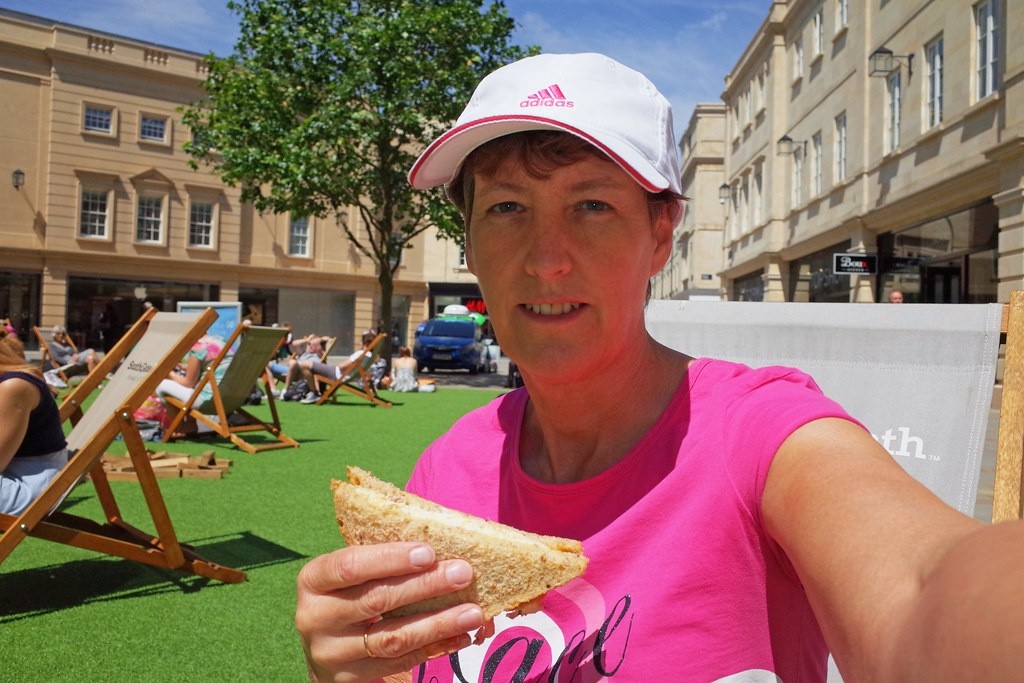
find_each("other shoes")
[300,391,321,404]
[279,390,287,400]
[104,372,113,380]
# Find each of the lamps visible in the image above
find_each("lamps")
[868,45,914,76]
[777,135,808,161]
[719,183,738,199]
[11,169,25,189]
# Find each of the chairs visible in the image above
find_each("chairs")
[1,305,248,585]
[160,317,301,454]
[274,332,391,410]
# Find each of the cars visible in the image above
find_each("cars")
[412,316,491,376]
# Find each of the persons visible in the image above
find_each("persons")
[0,330,67,518]
[301,330,376,403]
[889,290,903,303]
[387,347,419,392]
[263,322,330,400]
[157,330,234,433]
[49,326,99,373]
[295,52,1024,683]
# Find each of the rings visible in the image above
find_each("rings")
[365,623,376,658]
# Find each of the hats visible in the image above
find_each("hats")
[407,53,683,197]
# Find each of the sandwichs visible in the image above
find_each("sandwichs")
[330,467,589,643]
